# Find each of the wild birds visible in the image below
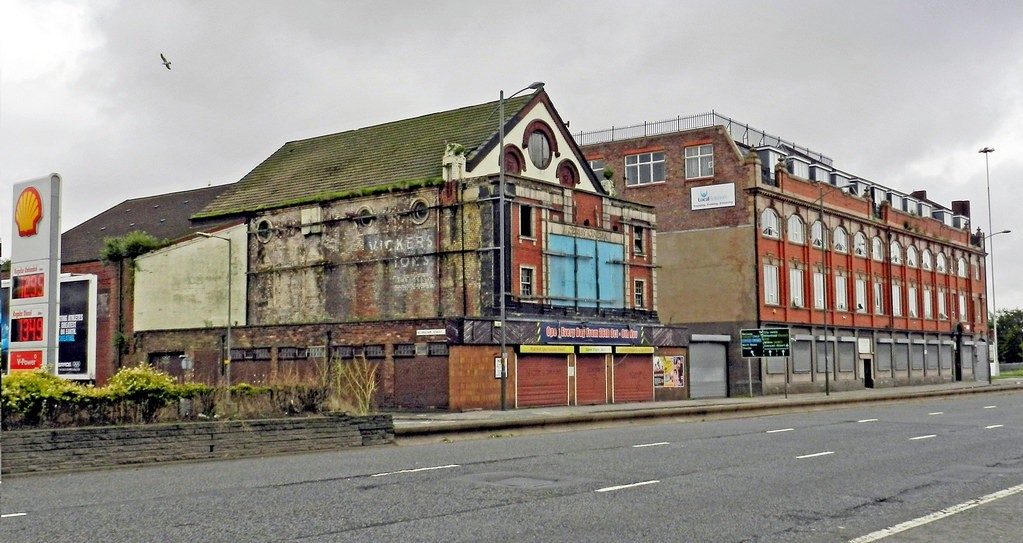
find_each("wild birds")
[160,54,171,71]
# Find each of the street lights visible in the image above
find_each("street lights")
[486,81,546,411]
[968,229,1011,380]
[806,182,857,396]
[195,231,233,409]
[978,147,998,378]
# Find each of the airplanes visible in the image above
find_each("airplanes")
[159,53,173,71]
